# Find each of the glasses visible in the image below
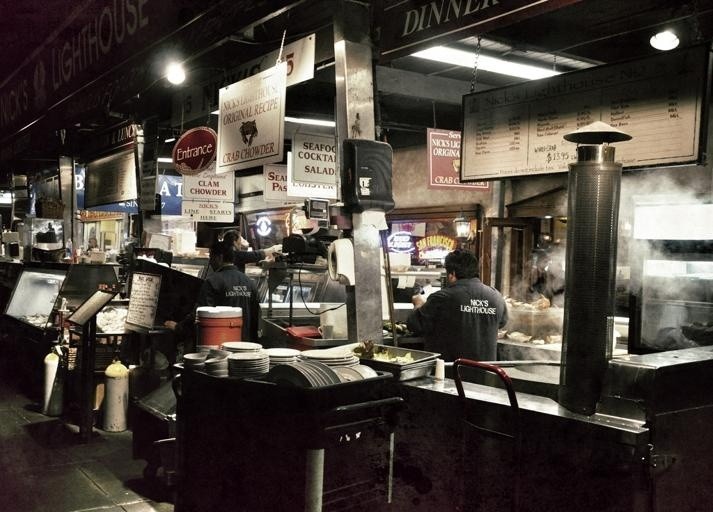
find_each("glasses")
[650,26,681,51]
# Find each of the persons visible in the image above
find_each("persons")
[524,252,548,298]
[401,248,508,387]
[161,240,260,344]
[542,272,566,309]
[222,227,282,274]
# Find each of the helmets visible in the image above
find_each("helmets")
[220,340,379,388]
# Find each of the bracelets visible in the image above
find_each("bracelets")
[498,330,533,342]
[183,348,233,378]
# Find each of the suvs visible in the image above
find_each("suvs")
[195,306,243,346]
